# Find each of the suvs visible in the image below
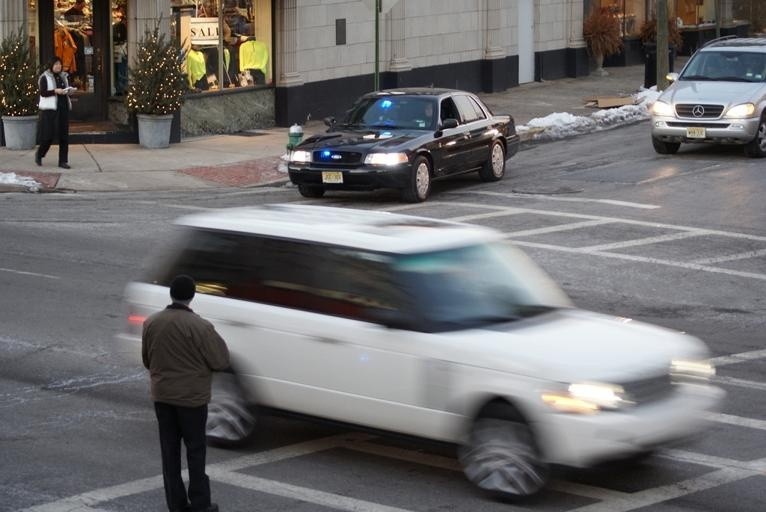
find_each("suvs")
[112,203,720,502]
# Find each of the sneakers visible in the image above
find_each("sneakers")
[191,501,220,512]
[33,151,71,169]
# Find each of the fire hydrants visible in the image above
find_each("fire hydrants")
[286,123,304,150]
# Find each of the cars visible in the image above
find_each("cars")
[288,87,520,203]
[651,34,766,158]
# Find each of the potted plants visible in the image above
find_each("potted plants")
[119,9,195,150]
[0,25,50,151]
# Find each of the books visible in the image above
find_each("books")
[62,86,78,98]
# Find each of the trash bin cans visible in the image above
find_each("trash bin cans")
[644,43,673,88]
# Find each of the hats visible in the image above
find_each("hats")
[166,275,197,301]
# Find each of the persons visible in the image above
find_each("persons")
[415,101,432,122]
[63,1,85,24]
[141,274,230,512]
[33,55,72,171]
